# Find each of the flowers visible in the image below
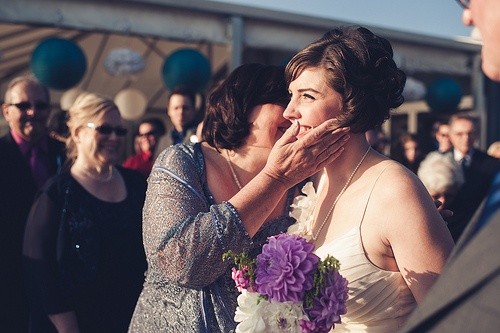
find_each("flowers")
[223,233,350,333]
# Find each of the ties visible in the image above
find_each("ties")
[460,157,467,168]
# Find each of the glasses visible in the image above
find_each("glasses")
[450,128,473,136]
[454,0,470,10]
[81,121,128,136]
[6,102,49,110]
[139,132,155,137]
[437,131,448,137]
